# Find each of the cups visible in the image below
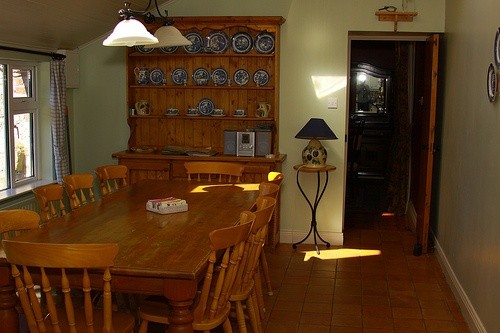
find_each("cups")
[234,110,246,115]
[167,108,179,114]
[187,108,199,114]
[213,109,224,115]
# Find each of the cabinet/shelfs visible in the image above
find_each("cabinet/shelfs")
[125,16,286,156]
[349,118,396,216]
[113,152,287,249]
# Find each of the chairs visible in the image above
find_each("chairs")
[0,162,284,333]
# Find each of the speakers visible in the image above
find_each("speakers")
[224,130,237,156]
[256,131,273,157]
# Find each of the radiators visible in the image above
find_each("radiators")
[0,197,60,236]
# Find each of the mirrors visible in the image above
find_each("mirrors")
[351,61,395,118]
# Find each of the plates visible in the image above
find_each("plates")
[212,68,227,85]
[486,32,500,101]
[232,115,248,118]
[198,99,214,115]
[164,114,179,116]
[233,69,250,85]
[193,68,210,85]
[149,69,164,85]
[183,32,205,54]
[253,69,269,86]
[160,46,179,54]
[185,114,202,117]
[211,115,226,117]
[205,30,230,54]
[171,68,188,85]
[254,32,275,54]
[231,31,253,54]
[135,45,155,54]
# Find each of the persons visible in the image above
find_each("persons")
[356,80,370,111]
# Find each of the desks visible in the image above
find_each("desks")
[0,177,262,333]
[292,164,336,255]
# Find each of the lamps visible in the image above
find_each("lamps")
[102,0,194,48]
[294,117,339,168]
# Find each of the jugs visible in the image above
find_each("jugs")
[254,102,271,118]
[133,66,150,86]
[135,99,151,115]
